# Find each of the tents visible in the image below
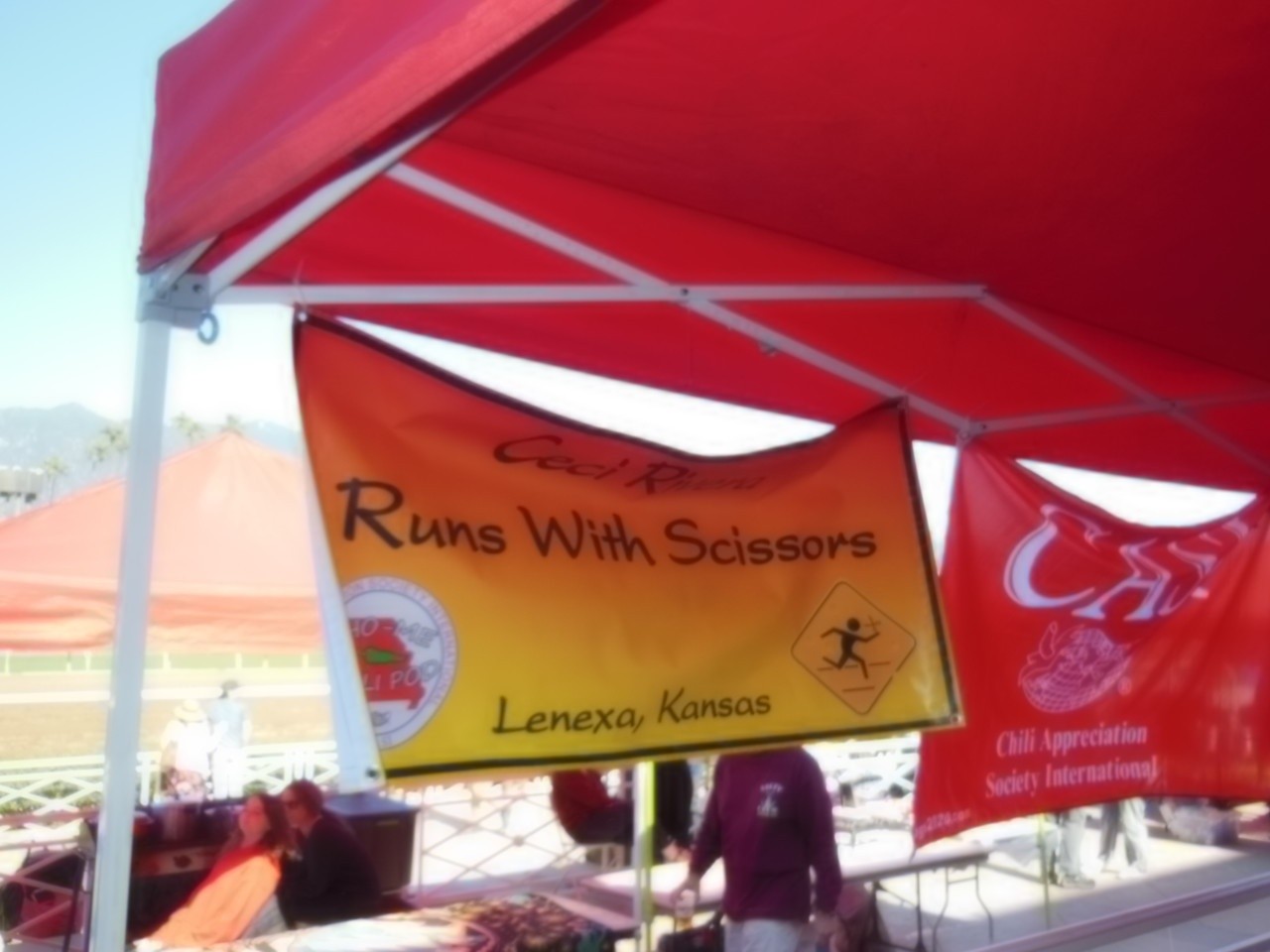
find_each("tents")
[88,0,1270,952]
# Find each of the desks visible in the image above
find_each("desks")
[167,889,644,952]
[584,828,994,952]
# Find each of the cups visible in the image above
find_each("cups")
[673,898,693,933]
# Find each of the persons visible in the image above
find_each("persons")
[211,741,1148,952]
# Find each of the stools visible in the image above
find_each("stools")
[583,842,623,874]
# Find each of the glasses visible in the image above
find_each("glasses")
[280,800,297,810]
